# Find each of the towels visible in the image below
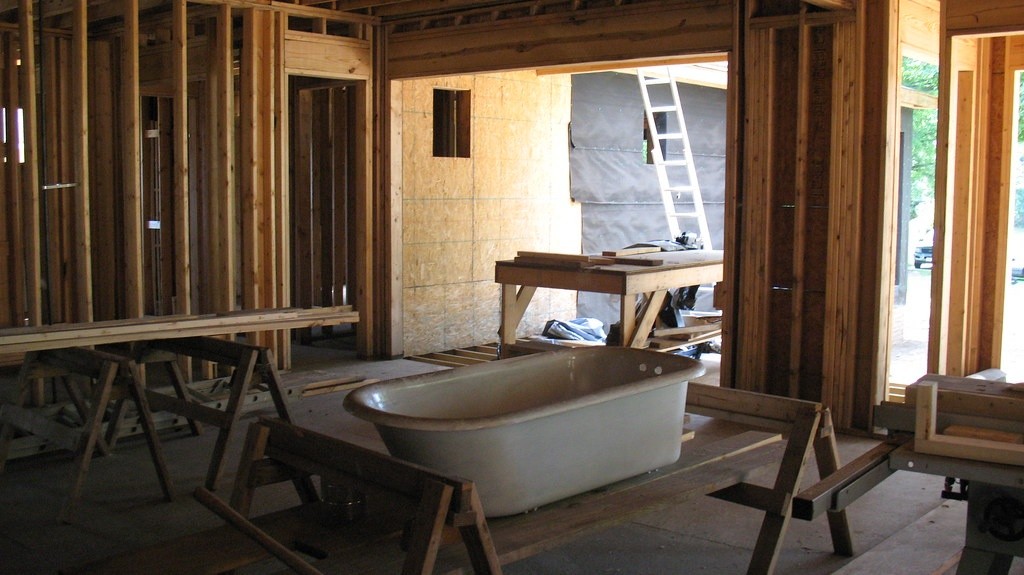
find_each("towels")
[530,316,608,349]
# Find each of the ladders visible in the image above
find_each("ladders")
[637,65,713,252]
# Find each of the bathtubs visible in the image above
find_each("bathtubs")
[341,343,708,521]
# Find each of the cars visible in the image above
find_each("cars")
[915,227,933,269]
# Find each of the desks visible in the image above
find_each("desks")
[494,247,726,354]
[889,438,1024,575]
